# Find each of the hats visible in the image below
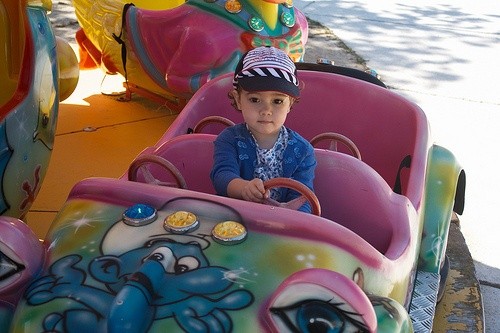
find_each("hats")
[231,45,301,100]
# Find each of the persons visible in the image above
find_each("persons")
[210,45,318,214]
